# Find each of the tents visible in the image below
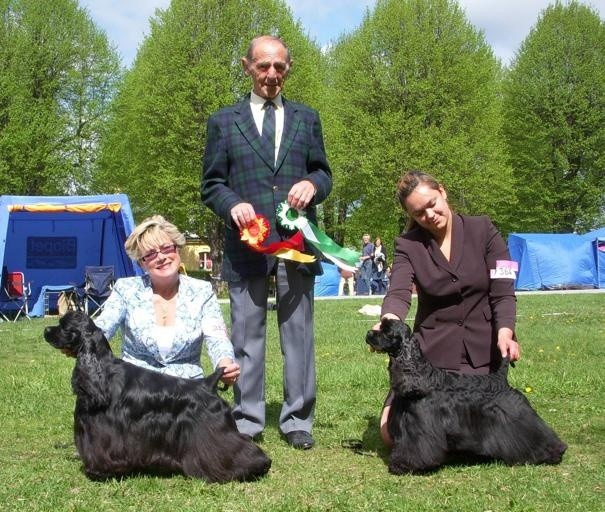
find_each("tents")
[502,221,605,291]
[0,193,148,320]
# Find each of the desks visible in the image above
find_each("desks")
[42,286,76,318]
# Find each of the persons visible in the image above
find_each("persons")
[357,233,376,295]
[56,214,242,387]
[337,245,358,298]
[198,35,336,451]
[361,168,523,444]
[370,238,389,295]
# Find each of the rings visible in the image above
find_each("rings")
[232,377,237,382]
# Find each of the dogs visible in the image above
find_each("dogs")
[43,310,272,485]
[364,316,568,476]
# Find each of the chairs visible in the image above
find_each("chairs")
[83,265,114,318]
[0,272,31,322]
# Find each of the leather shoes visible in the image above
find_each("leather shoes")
[286,431,315,450]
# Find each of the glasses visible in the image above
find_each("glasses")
[142,244,177,263]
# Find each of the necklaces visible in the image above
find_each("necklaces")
[153,288,177,320]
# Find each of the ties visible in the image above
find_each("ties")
[262,102,276,169]
